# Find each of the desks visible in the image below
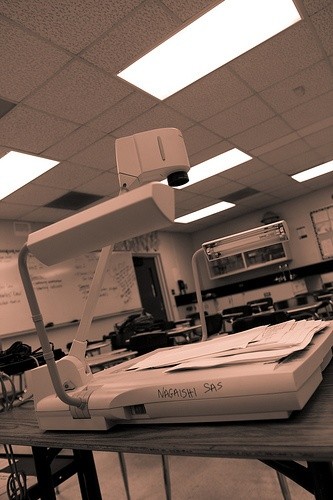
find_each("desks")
[65,320,202,374]
[1,360,333,500]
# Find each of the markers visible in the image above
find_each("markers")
[69,319,80,322]
[45,321,54,329]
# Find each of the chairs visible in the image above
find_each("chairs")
[179,280,332,338]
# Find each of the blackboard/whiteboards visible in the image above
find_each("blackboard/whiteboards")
[0,247,144,338]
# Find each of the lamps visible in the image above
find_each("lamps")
[18,181,175,403]
[193,218,289,341]
